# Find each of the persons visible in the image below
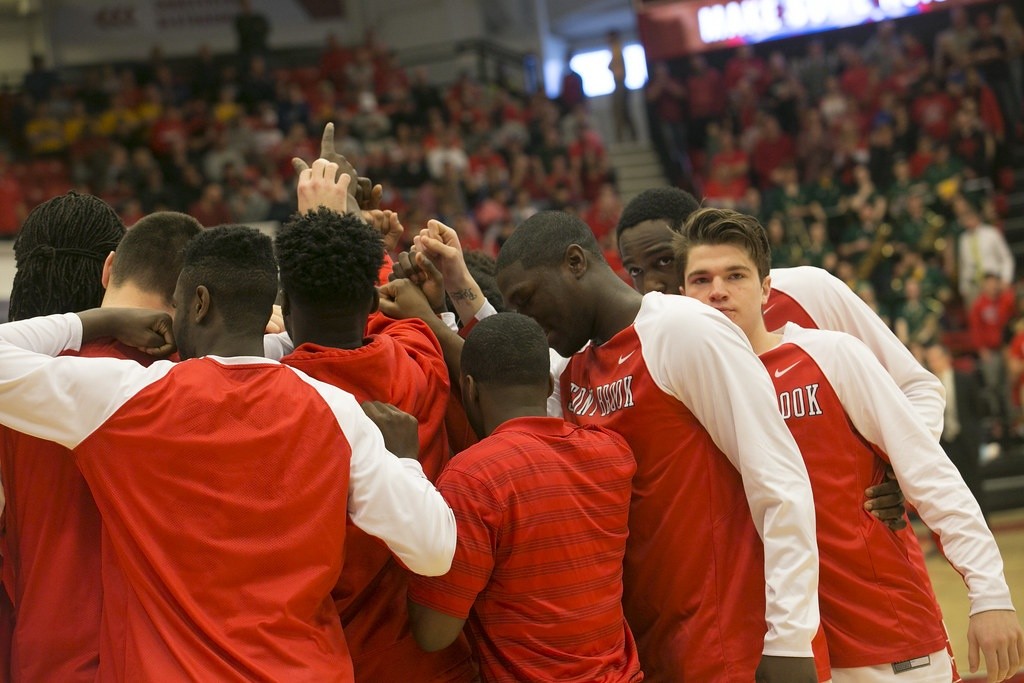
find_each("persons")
[969,271,1019,444]
[0,122,1024,683]
[376,278,645,683]
[0,0,1024,511]
[955,204,1013,306]
[603,31,640,143]
[0,223,458,683]
